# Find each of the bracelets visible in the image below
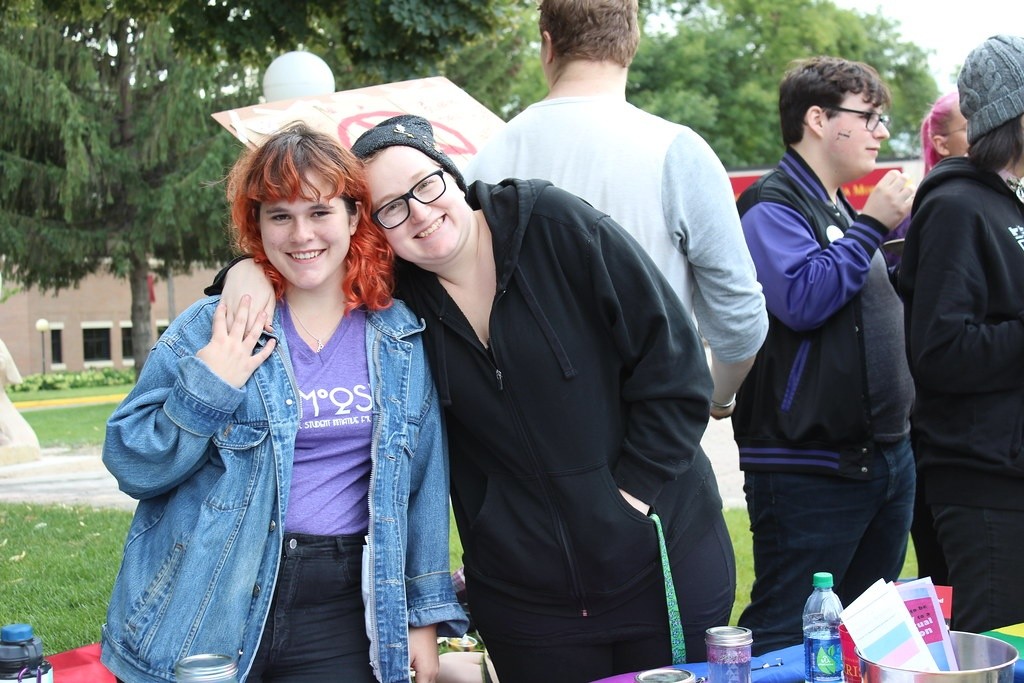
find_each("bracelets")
[711,393,736,408]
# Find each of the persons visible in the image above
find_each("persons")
[460,1,769,420]
[729,57,915,658]
[881,90,969,289]
[101,122,469,683]
[899,34,1024,634]
[204,116,736,683]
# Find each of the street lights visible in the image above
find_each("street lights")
[36,318,49,375]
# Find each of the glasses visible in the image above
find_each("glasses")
[371,168,446,229]
[838,107,890,132]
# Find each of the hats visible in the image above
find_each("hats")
[349,114,468,200]
[957,34,1024,143]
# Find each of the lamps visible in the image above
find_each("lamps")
[263,49,337,103]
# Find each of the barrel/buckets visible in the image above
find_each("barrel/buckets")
[854,630,1020,683]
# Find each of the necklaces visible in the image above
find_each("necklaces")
[287,300,341,352]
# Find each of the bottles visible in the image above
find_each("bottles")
[803,572,843,683]
[0,624,43,670]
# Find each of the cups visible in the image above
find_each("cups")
[838,624,863,683]
